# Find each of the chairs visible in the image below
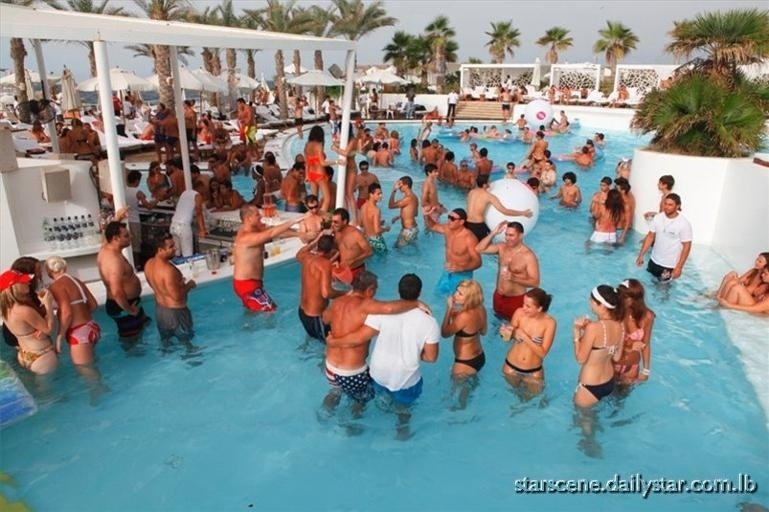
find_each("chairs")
[0,89,438,147]
[464,82,644,108]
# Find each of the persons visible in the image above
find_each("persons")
[0,74,769,449]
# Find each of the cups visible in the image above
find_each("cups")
[502,323,514,342]
[331,264,354,286]
[33,286,48,299]
[499,262,508,273]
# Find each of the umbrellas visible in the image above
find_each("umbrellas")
[1,63,412,118]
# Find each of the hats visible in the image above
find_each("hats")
[0,271,35,292]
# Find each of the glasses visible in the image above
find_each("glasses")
[447,214,461,222]
[307,204,318,210]
